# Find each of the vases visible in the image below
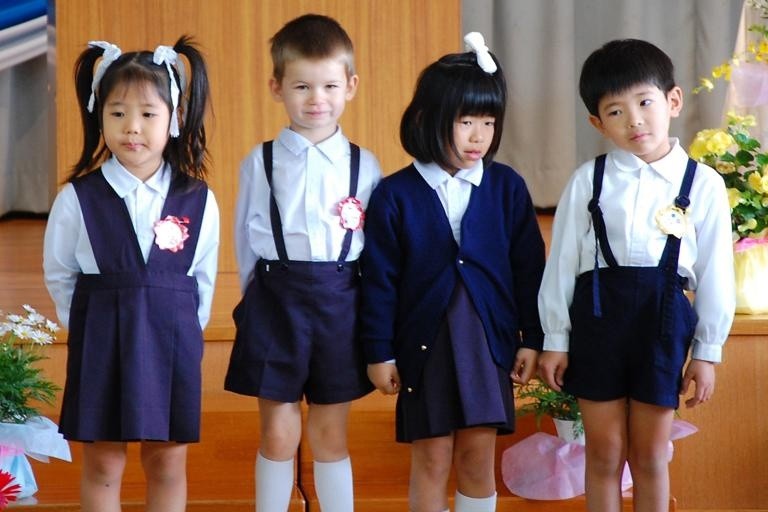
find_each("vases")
[550,415,585,446]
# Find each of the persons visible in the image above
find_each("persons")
[224,9,386,512]
[356,47,548,512]
[534,35,739,512]
[39,33,221,512]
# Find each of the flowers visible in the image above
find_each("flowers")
[682,1,768,246]
[511,375,585,441]
[0,299,63,425]
